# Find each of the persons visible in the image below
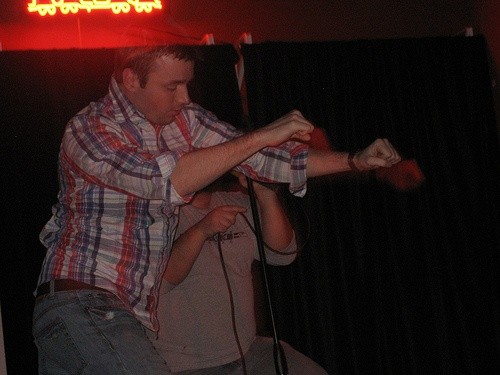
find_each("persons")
[30,44,402,375]
[143,170,328,375]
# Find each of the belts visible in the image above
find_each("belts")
[36,279,112,298]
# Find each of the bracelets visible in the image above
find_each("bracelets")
[347,147,362,172]
